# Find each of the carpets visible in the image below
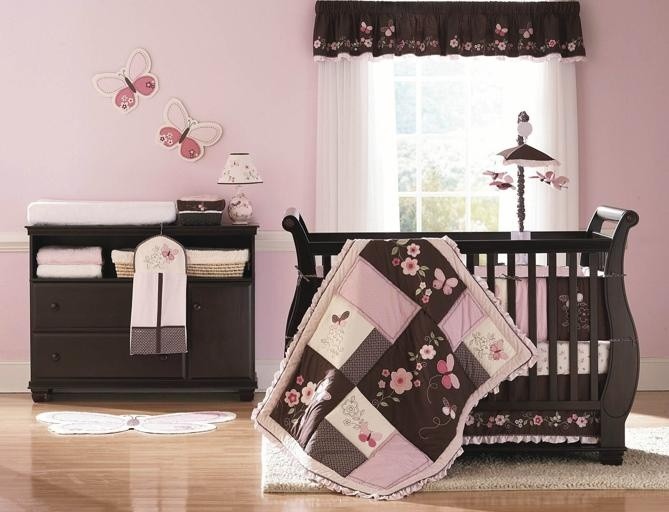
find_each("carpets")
[257,387,669,494]
[33,408,237,438]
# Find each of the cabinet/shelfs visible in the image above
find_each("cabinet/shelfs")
[24,222,260,402]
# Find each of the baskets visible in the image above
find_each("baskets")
[110,247,250,279]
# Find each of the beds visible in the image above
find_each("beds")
[281,205,640,471]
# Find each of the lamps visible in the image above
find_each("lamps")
[218,151,263,226]
[495,109,561,232]
